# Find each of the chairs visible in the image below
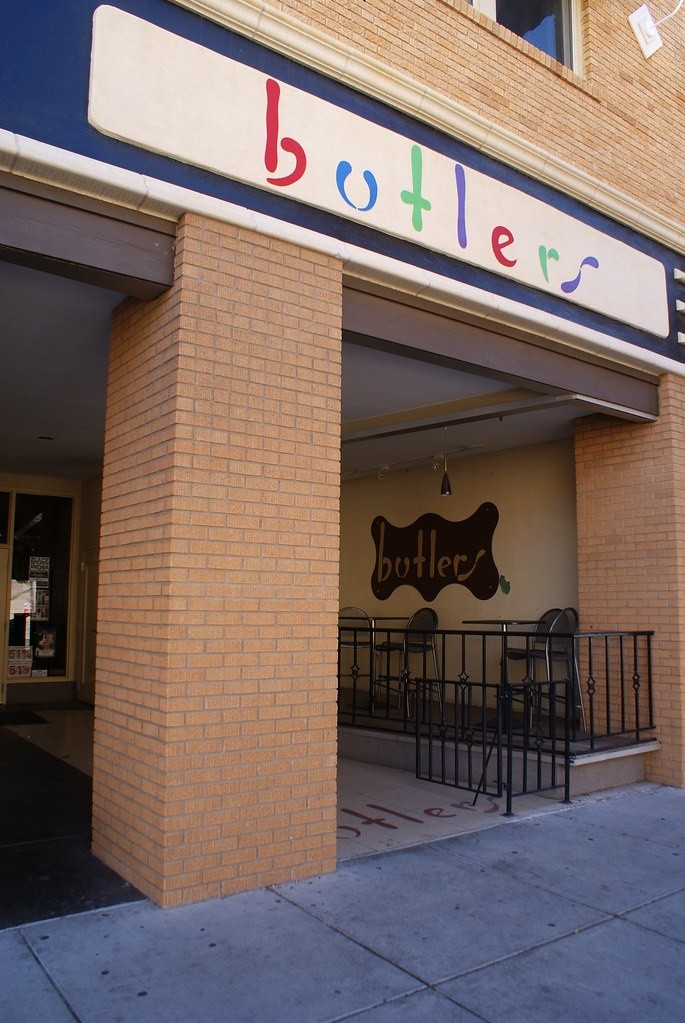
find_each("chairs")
[378,607,442,719]
[506,607,587,734]
[338,607,370,647]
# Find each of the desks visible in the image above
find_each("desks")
[461,619,547,730]
[338,616,411,710]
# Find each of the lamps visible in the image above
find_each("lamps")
[441,454,452,496]
